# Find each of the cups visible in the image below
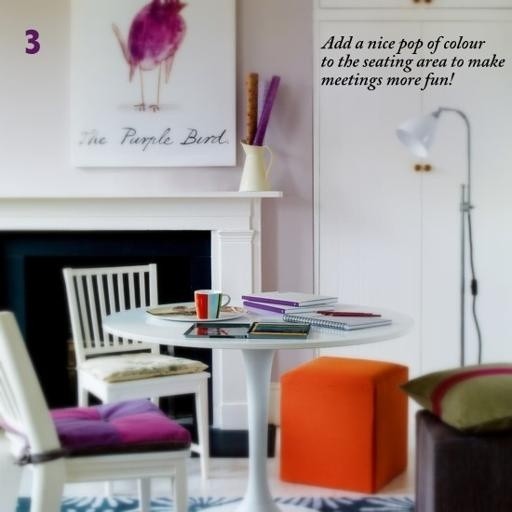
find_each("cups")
[193,289,230,319]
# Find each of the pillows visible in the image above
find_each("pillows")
[395,356,511,433]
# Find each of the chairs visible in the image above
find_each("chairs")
[0,309,197,512]
[58,259,221,482]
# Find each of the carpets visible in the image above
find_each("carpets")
[2,485,421,512]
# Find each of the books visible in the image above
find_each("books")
[242,301,336,314]
[282,311,392,332]
[241,291,340,307]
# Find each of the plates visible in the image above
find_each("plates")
[153,303,249,322]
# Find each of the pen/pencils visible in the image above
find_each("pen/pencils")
[316,311,381,317]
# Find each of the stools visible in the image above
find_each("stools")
[411,396,512,511]
[276,351,412,493]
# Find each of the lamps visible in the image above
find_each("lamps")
[393,97,490,371]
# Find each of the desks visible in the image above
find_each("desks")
[99,289,421,512]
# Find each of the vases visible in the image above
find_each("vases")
[237,137,277,197]
